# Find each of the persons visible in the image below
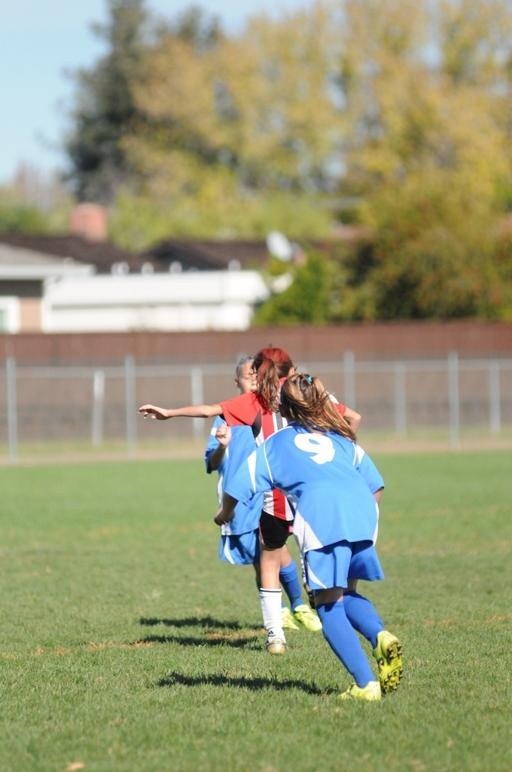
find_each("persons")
[205,356,323,632]
[137,347,361,654]
[214,373,404,704]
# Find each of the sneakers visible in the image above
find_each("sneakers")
[372,630,403,697]
[281,604,324,631]
[337,680,381,701]
[266,637,287,655]
